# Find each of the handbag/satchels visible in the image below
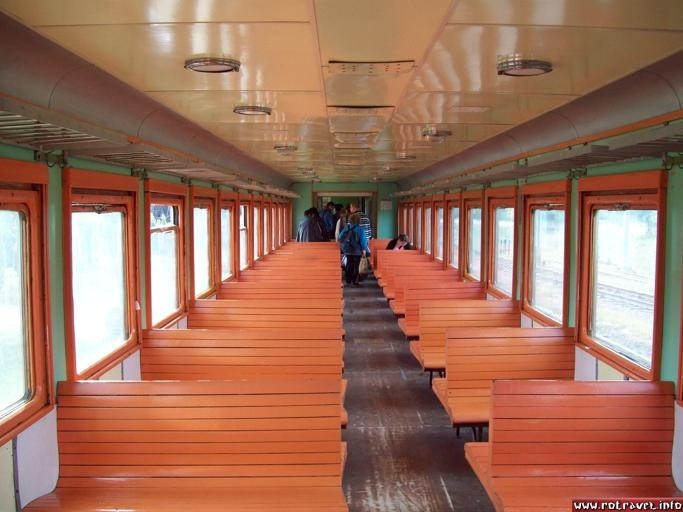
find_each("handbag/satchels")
[358,255,369,275]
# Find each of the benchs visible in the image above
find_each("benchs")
[370,239,683,512]
[19,239,350,512]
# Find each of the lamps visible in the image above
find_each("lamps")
[497,60,552,77]
[184,57,241,73]
[232,106,271,116]
[422,128,454,144]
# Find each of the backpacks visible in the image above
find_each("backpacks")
[342,225,363,256]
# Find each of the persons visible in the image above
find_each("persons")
[346,201,372,282]
[337,214,368,288]
[295,209,322,243]
[385,234,410,250]
[310,201,350,243]
